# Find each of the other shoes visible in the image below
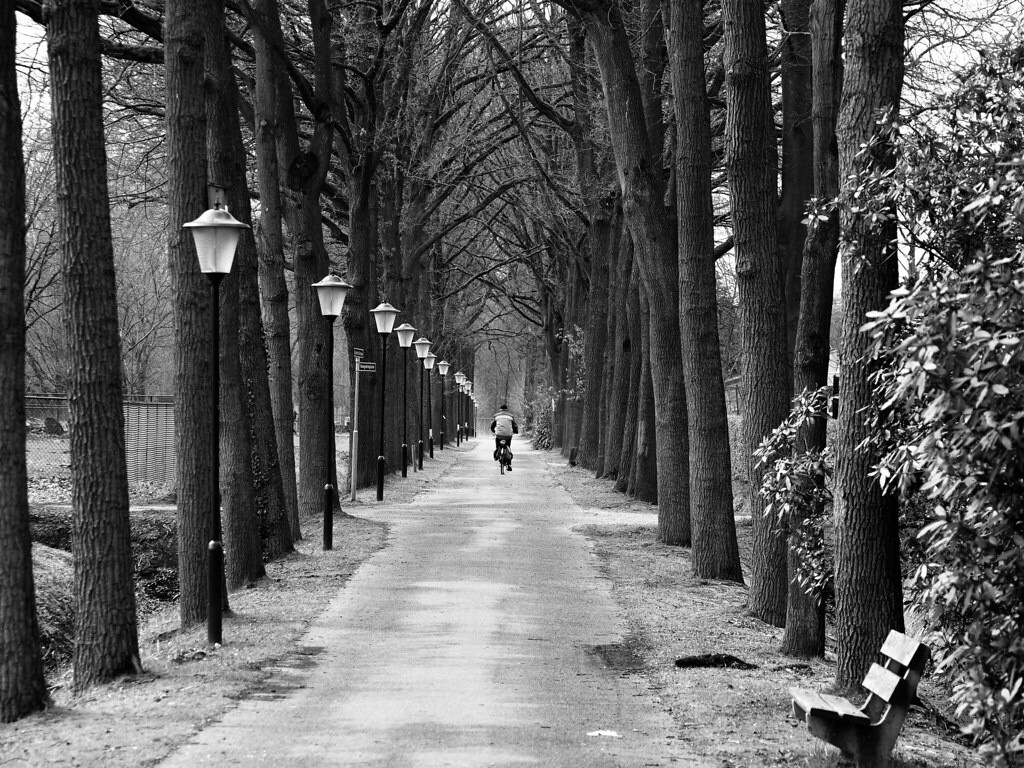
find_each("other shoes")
[508,468,512,471]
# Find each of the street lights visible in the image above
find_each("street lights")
[368,298,403,501]
[414,337,433,470]
[424,351,438,460]
[454,371,479,447]
[184,197,250,644]
[310,265,355,551]
[394,322,418,478]
[437,360,451,450]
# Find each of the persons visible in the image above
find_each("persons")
[490,404,519,471]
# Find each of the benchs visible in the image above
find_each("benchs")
[790,629,932,768]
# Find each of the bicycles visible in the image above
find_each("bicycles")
[495,440,513,474]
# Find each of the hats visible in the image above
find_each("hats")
[500,405,508,409]
